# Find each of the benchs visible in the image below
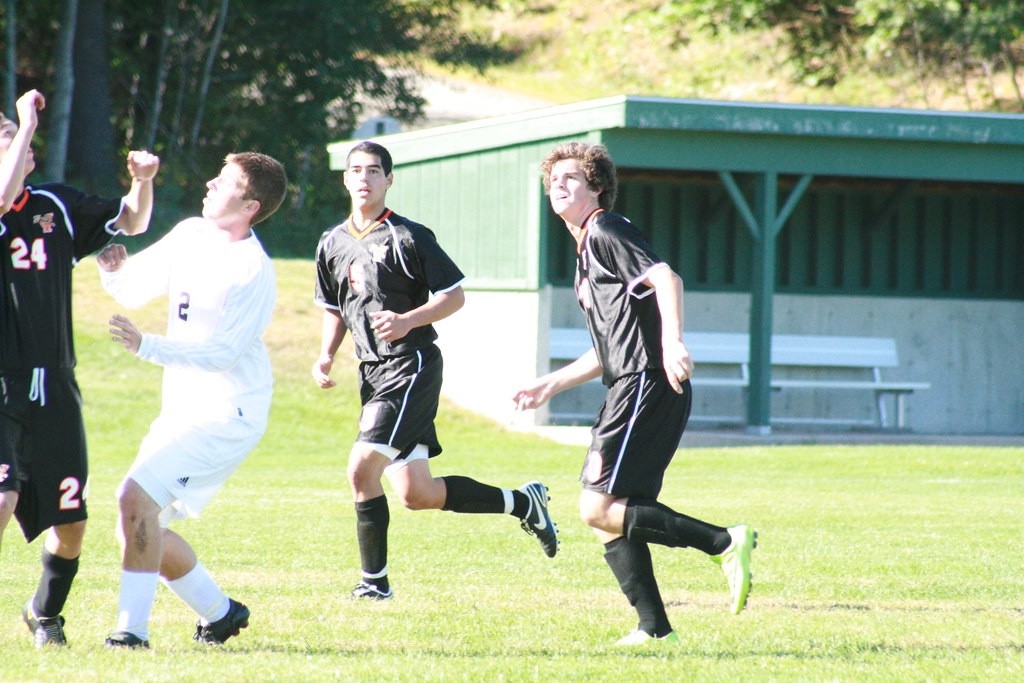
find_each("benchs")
[549,328,932,428]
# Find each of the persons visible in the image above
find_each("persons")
[97,153,287,648]
[509,138,759,649]
[313,142,560,601]
[0,88,158,649]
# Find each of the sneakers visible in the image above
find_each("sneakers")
[709,525,758,615]
[193,597,250,644]
[104,632,147,648]
[613,629,679,644]
[515,482,561,558]
[20,601,67,647]
[343,583,392,599]
